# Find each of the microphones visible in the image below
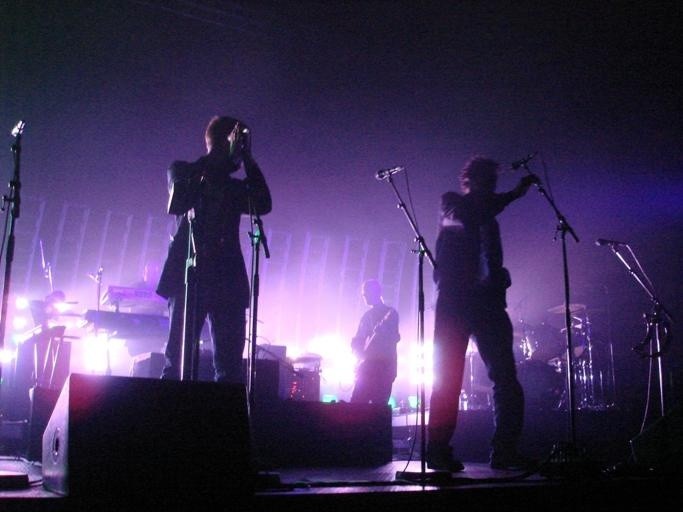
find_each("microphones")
[11,120,25,137]
[375,164,403,180]
[227,128,249,142]
[513,298,524,311]
[512,151,535,169]
[594,237,626,248]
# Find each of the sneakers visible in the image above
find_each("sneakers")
[426,442,463,472]
[489,442,538,471]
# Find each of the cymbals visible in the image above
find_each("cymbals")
[547,304,587,313]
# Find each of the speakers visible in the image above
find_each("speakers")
[43,374,248,494]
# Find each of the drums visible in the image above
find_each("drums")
[525,324,566,360]
[560,323,609,369]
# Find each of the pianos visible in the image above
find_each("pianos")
[80,286,210,340]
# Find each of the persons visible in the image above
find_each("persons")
[427,155,541,473]
[156,114,273,381]
[349,281,401,404]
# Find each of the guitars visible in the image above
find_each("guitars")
[356,308,391,365]
